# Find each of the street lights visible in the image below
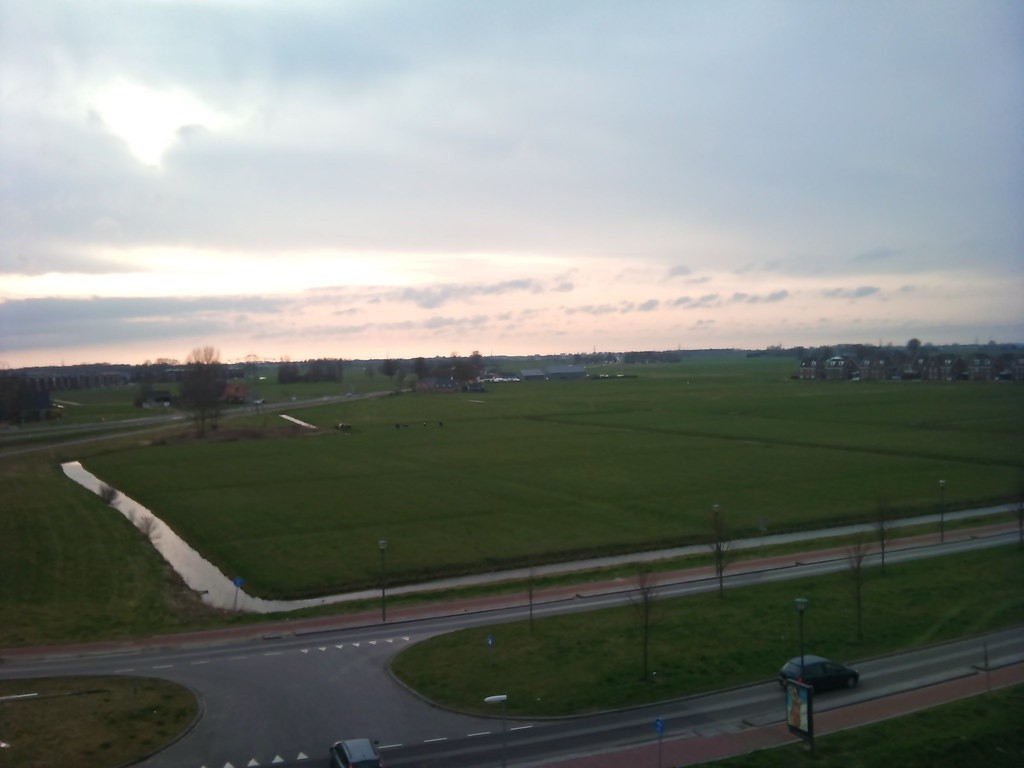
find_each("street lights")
[379,539,389,623]
[794,596,809,683]
[937,479,948,544]
[712,504,723,591]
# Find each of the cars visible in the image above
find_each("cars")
[329,737,384,768]
[779,654,860,692]
